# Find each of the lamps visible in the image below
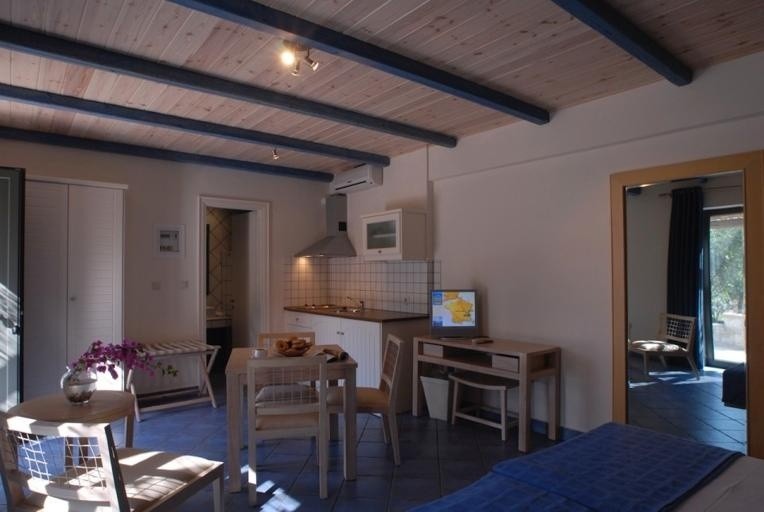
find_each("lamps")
[270,145,281,161]
[290,46,320,78]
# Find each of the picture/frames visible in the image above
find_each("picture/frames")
[151,223,185,260]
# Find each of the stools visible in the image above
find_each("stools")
[447,370,519,441]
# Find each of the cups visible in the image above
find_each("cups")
[253,349,269,359]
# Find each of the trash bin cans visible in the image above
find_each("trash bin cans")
[420,377,463,421]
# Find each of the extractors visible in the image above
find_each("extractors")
[293,194,358,258]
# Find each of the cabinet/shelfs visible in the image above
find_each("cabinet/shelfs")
[23,176,128,401]
[359,208,426,261]
[120,338,221,422]
[283,310,429,419]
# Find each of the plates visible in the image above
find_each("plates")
[248,356,271,360]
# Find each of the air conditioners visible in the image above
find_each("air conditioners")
[331,163,382,194]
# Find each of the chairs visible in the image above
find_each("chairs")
[246,332,405,508]
[0,410,223,511]
[627,312,700,380]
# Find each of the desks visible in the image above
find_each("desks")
[224,344,358,494]
[5,390,135,469]
[412,335,561,453]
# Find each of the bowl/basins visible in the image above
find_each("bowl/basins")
[274,347,311,357]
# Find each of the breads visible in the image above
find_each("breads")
[275,335,306,353]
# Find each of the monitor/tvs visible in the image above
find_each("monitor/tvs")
[428,288,481,341]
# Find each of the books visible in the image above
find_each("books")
[302,345,349,363]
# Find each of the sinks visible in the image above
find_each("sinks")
[331,309,361,313]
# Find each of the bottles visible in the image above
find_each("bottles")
[215,302,225,317]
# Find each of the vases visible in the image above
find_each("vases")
[59,366,98,406]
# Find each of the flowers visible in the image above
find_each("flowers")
[65,336,179,382]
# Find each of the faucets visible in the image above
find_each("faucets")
[347,296,364,309]
